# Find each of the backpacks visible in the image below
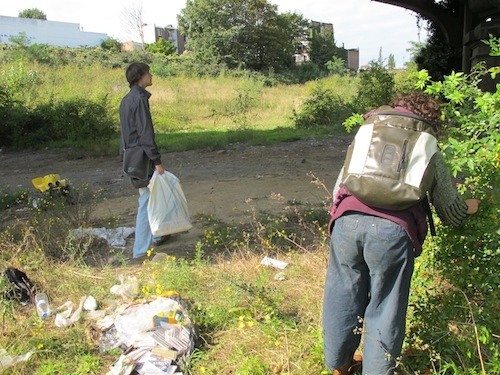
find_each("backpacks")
[341,105,436,211]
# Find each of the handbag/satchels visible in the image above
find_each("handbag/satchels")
[146,169,192,236]
[123,148,155,188]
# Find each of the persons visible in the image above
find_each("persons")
[119,62,172,261]
[323,93,480,375]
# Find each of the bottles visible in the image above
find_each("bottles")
[35,293,50,319]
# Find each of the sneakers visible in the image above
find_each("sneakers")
[332,353,362,375]
[153,235,172,246]
